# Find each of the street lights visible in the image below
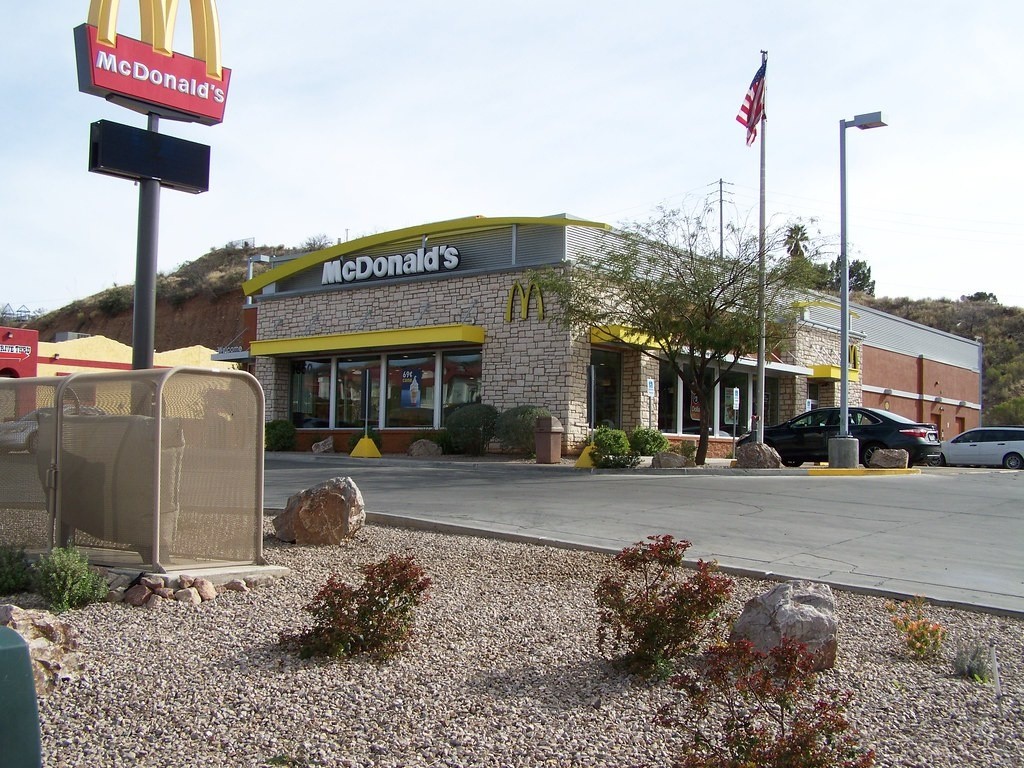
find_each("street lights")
[825,112,886,468]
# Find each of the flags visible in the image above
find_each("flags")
[735,51,768,148]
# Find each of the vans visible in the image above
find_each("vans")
[926,426,1024,469]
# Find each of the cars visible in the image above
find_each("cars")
[683,426,730,437]
[734,406,942,468]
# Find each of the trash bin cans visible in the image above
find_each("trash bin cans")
[535,416,564,464]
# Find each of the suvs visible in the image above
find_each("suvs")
[0,406,108,453]
